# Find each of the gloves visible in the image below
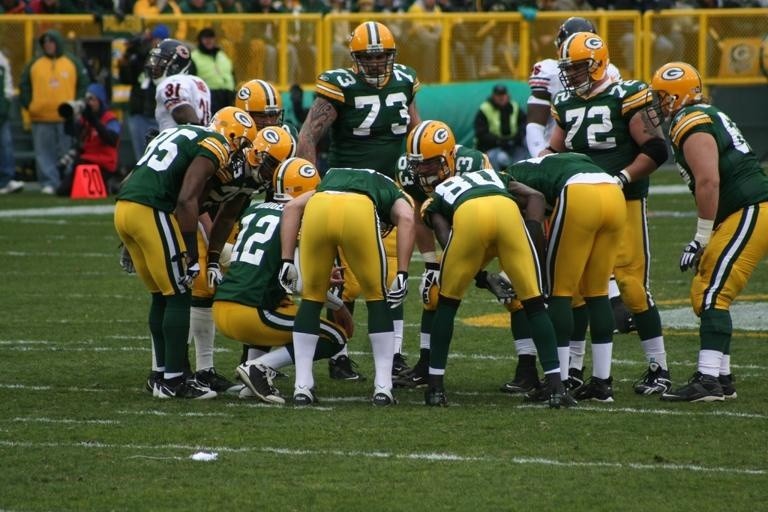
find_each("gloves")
[486,273,516,305]
[680,241,701,272]
[386,271,407,309]
[120,246,137,274]
[206,252,223,288]
[419,262,442,304]
[179,263,200,287]
[278,259,297,294]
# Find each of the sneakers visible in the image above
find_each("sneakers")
[11,182,22,190]
[0,186,10,195]
[43,186,54,195]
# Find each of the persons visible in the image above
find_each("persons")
[644,61,768,401]
[190,78,297,392]
[298,22,419,385]
[120,27,169,161]
[540,33,671,394]
[1,0,768,85]
[1,53,24,195]
[278,168,416,406]
[115,107,258,398]
[20,29,87,193]
[148,39,211,129]
[58,83,121,196]
[398,118,541,391]
[423,170,578,406]
[475,84,528,169]
[213,157,354,403]
[504,150,630,403]
[190,28,237,118]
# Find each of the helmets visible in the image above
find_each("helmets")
[349,22,396,88]
[210,79,321,200]
[646,62,702,129]
[150,38,193,83]
[407,120,456,191]
[556,17,596,49]
[558,32,609,97]
